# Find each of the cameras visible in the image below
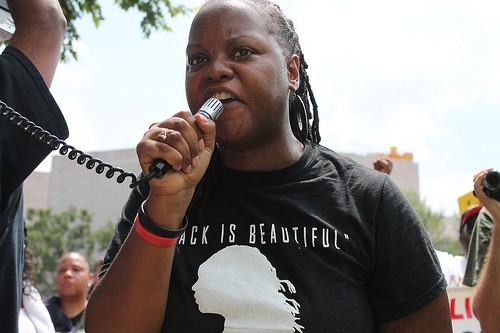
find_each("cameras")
[473,171,500,200]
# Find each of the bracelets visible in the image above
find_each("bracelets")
[133,201,188,246]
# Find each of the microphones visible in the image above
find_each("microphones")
[149,97,224,178]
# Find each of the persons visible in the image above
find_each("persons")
[191,245,305,333]
[459,168,500,333]
[0,0,104,333]
[83,0,453,333]
